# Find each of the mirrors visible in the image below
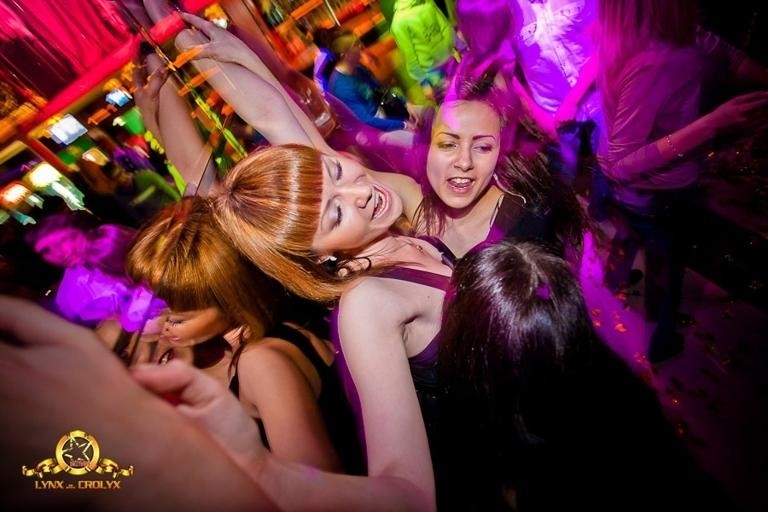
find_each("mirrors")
[2,0,419,476]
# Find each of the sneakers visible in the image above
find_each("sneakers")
[609,268,695,365]
